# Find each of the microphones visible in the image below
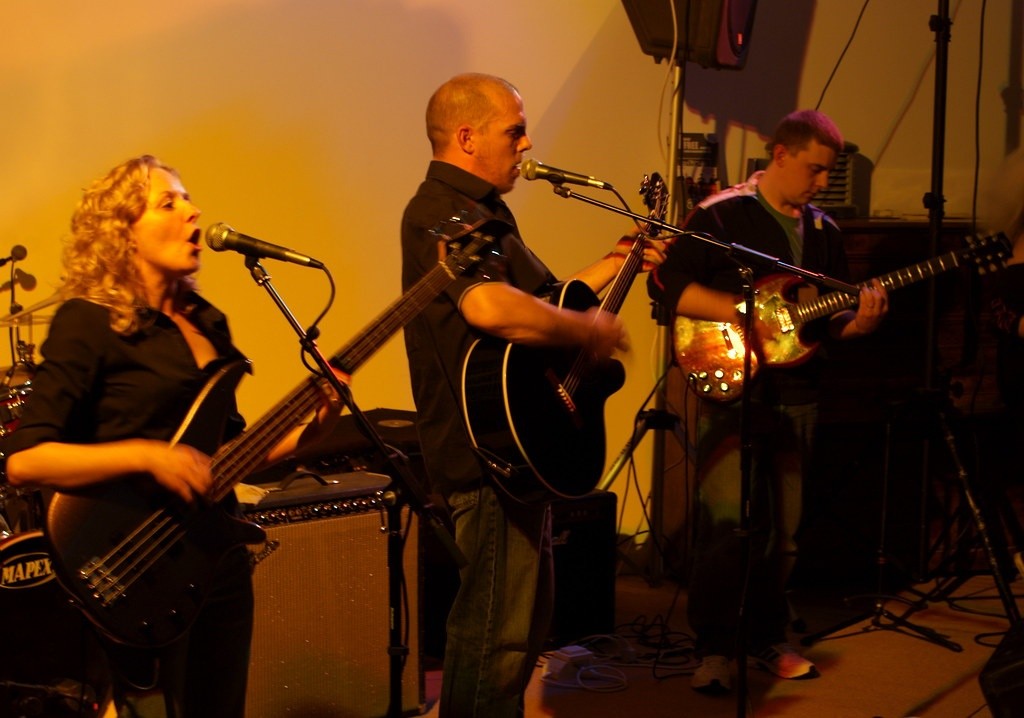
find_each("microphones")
[11,245,27,260]
[520,158,613,190]
[205,222,324,269]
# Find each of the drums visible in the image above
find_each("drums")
[0,527,98,710]
[0,386,57,499]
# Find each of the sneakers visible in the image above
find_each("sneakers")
[747,643,816,679]
[690,655,731,692]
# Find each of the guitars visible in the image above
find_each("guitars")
[672,227,1018,408]
[43,208,518,656]
[458,167,675,512]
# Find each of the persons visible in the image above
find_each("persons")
[4,154,352,716]
[401,73,667,716]
[646,109,890,693]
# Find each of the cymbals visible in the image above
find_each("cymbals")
[8,288,65,321]
[0,313,53,328]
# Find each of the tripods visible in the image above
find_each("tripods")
[799,0,1024,652]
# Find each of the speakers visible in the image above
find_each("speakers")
[529,489,618,644]
[242,472,420,718]
[622,0,758,70]
